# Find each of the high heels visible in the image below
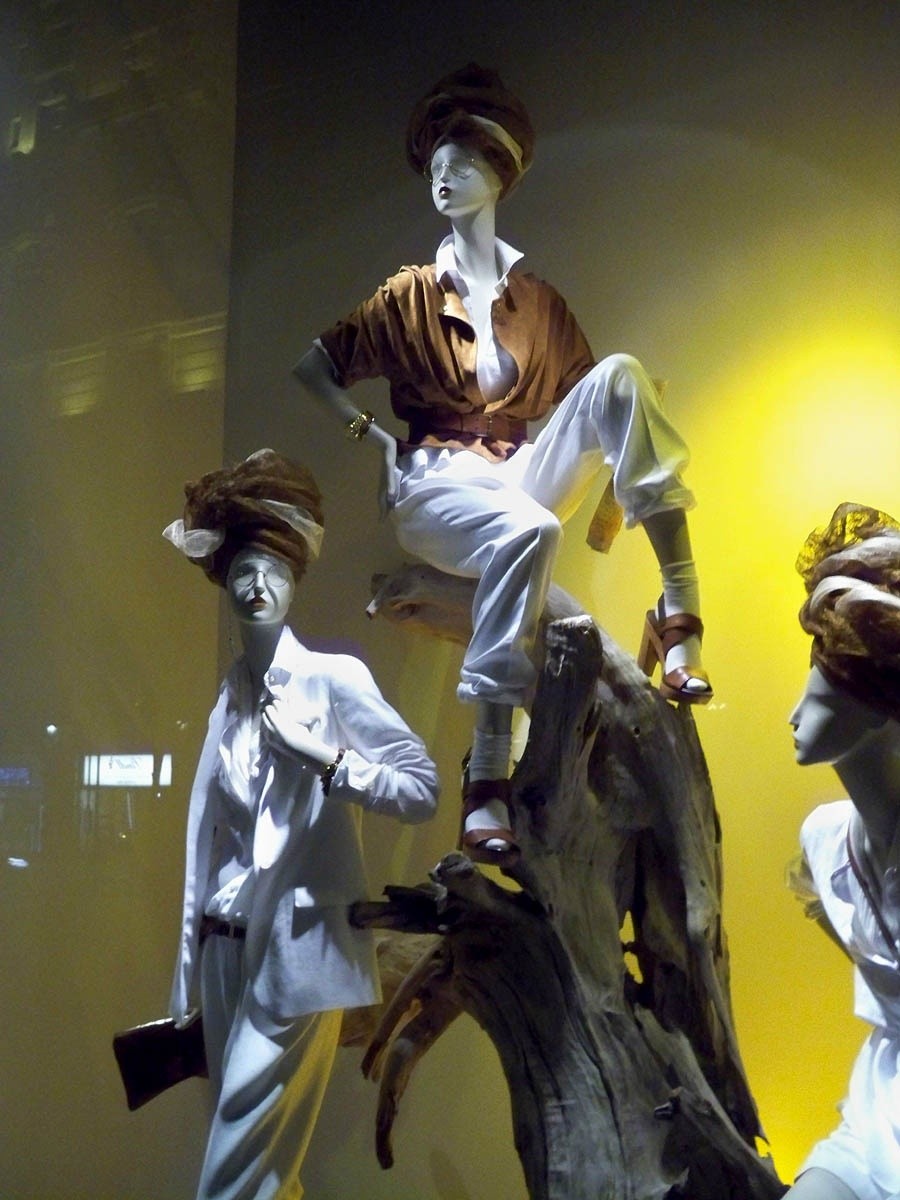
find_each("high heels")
[459,746,520,867]
[637,592,714,704]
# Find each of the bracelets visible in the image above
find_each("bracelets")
[341,409,375,443]
[320,748,345,797]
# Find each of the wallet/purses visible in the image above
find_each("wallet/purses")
[113,1006,208,1113]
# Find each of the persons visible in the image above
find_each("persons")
[777,501,900,1200]
[170,450,435,1200]
[287,64,714,865]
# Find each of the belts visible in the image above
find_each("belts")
[199,912,246,948]
[408,408,529,446]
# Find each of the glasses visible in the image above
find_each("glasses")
[226,562,294,587]
[423,155,494,186]
